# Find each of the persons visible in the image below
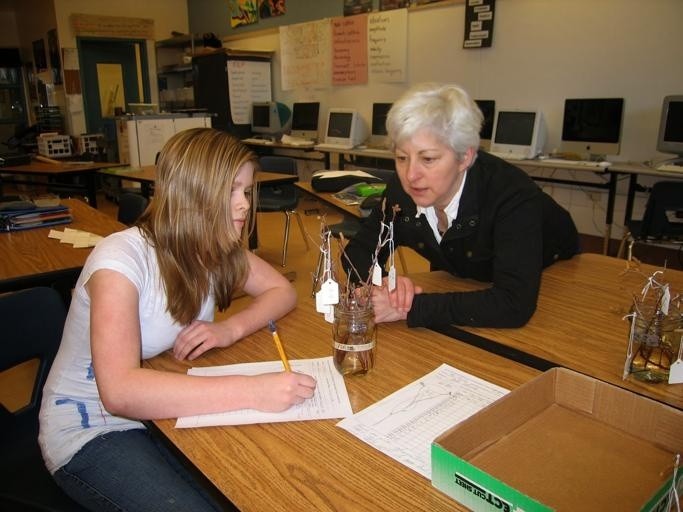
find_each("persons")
[38,128,297,512]
[341,80,579,328]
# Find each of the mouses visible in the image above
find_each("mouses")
[599,161,612,168]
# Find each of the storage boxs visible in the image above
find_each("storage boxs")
[430,366,682,512]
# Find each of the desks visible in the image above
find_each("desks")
[242,136,330,170]
[611,151,682,265]
[294,171,381,218]
[508,152,618,255]
[1,194,132,284]
[408,253,683,410]
[141,273,544,512]
[314,139,398,171]
[100,164,297,250]
[0,161,130,215]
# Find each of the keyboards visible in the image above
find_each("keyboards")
[542,158,597,166]
[315,143,354,149]
[657,164,683,174]
[244,139,270,143]
[362,148,393,155]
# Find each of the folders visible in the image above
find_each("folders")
[0,207,72,231]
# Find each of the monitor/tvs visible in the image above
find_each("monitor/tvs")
[371,101,393,136]
[561,97,626,162]
[324,108,369,145]
[490,108,546,159]
[657,95,683,164]
[474,98,496,141]
[291,101,320,140]
[251,102,281,133]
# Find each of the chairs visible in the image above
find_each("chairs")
[311,163,407,299]
[1,286,88,510]
[257,153,311,266]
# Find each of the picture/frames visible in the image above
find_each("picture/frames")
[409,0,465,12]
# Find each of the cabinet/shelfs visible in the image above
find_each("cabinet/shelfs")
[192,48,275,138]
[155,34,220,110]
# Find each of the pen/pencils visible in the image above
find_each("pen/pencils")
[267,319,290,372]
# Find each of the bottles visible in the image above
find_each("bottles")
[331,301,378,378]
[627,301,683,386]
